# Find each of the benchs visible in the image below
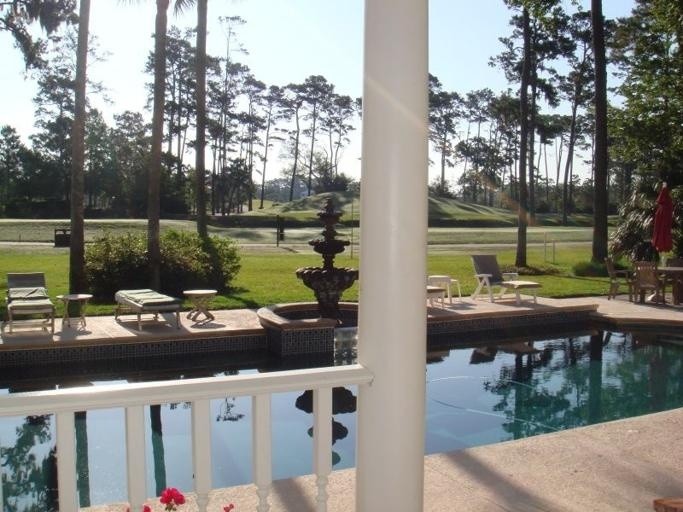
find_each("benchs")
[114,288,182,333]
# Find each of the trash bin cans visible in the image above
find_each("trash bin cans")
[55,229,71,247]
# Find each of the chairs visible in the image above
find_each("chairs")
[473,255,539,305]
[5,272,55,337]
[605,257,682,307]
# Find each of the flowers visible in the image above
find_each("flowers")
[121,486,235,512]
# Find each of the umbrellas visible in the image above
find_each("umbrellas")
[651,181,676,284]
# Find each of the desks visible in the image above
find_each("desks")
[56,292,94,336]
[183,290,219,322]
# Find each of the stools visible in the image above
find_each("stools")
[426,276,461,310]
